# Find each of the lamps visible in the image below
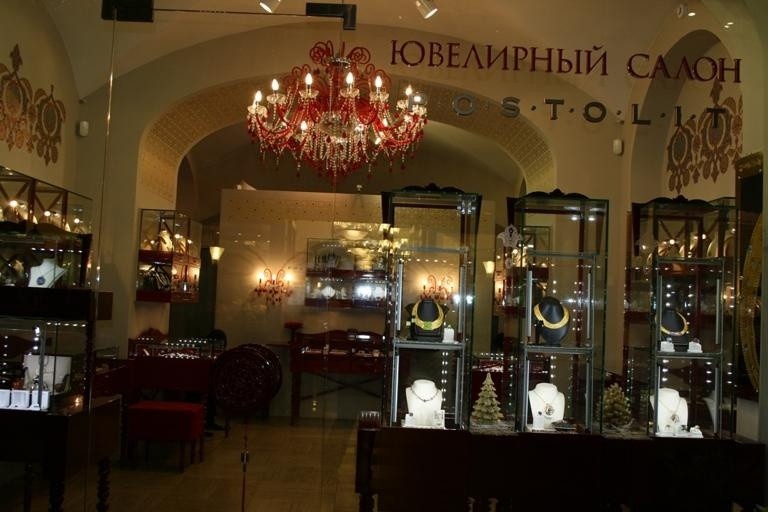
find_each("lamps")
[201,246,224,266]
[243,0,440,190]
[255,268,293,308]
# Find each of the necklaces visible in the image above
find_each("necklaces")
[654,311,689,336]
[37,265,60,286]
[411,299,445,331]
[411,385,438,402]
[534,303,570,329]
[658,397,681,422]
[533,389,560,416]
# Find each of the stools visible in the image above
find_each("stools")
[125,399,205,474]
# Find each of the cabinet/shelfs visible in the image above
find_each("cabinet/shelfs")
[136,208,203,306]
[70,364,128,402]
[130,334,226,428]
[290,183,768,512]
[1,164,122,512]
